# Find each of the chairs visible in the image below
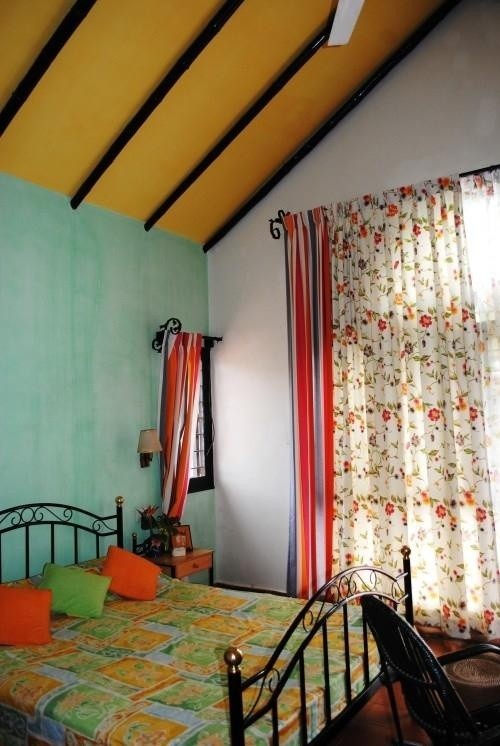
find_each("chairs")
[360,592,500,744]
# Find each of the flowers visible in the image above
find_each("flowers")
[139,505,180,551]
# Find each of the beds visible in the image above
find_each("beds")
[0,495,414,744]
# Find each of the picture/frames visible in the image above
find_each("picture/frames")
[169,525,192,555]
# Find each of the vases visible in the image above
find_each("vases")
[143,542,165,559]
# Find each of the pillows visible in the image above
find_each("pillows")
[0,544,157,645]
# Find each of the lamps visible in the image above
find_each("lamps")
[137,427,161,468]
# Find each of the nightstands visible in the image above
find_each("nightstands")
[142,550,215,587]
